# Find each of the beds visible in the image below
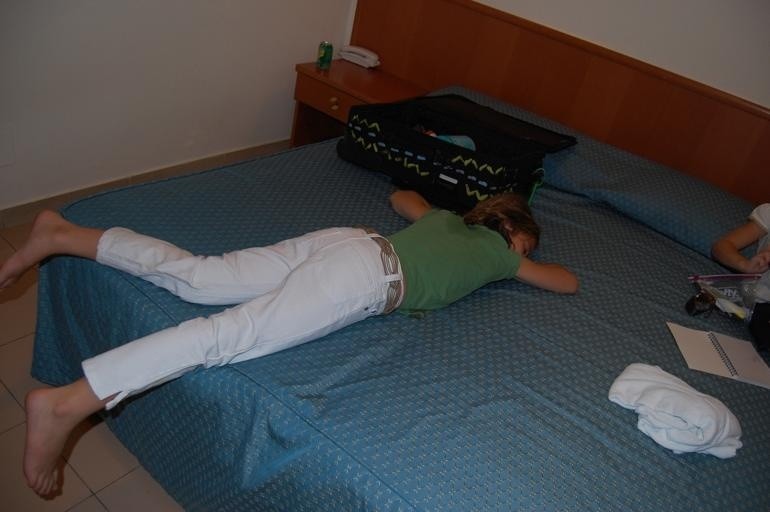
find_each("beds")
[31,0,770,512]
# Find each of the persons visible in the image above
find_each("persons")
[711,202,770,366]
[0,186,578,498]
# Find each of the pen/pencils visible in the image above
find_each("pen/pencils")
[698,282,746,319]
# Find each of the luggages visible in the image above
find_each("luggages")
[337,94,579,218]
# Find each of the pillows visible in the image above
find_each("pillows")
[596,162,770,259]
[426,85,649,200]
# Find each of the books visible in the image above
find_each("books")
[666,321,770,390]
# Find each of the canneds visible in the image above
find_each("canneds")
[317,40,333,69]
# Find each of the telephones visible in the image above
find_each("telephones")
[338,45,379,69]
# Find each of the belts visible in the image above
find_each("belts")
[359,222,403,313]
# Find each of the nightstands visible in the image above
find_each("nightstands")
[290,58,430,148]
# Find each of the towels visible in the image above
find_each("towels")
[608,362,743,461]
